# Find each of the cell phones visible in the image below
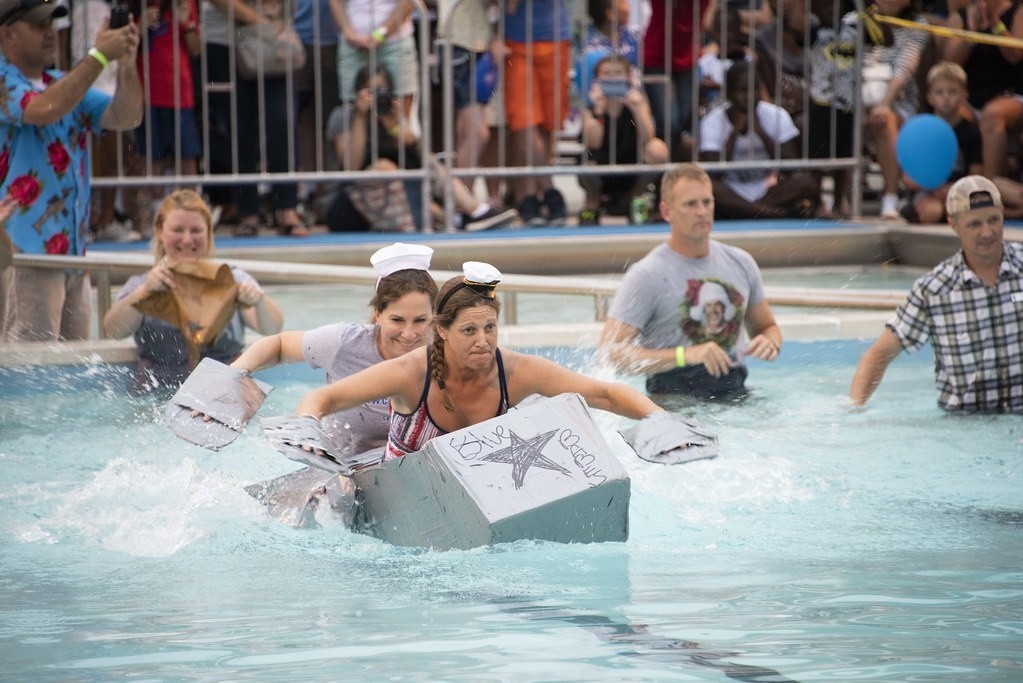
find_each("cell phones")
[600,78,631,98]
[109,6,129,29]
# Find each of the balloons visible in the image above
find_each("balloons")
[896,115,958,189]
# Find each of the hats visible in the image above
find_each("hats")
[946,174,1003,217]
[369,243,434,278]
[462,258,502,299]
[0,0,71,27]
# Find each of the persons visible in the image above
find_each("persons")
[297,261,687,463]
[0,0,143,343]
[104,189,283,383]
[190,242,438,458]
[47,0,1023,237]
[600,164,782,391]
[851,176,1023,415]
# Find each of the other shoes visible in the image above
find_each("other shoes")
[275,217,309,237]
[520,196,545,227]
[581,209,597,226]
[543,191,569,225]
[234,221,259,236]
[628,202,652,226]
[879,204,909,227]
[465,206,519,231]
[784,198,813,215]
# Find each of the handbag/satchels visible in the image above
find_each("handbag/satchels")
[239,27,307,80]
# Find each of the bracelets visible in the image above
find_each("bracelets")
[184,27,196,34]
[992,23,1005,33]
[388,125,400,135]
[378,26,388,37]
[676,346,684,368]
[372,30,384,42]
[593,112,604,119]
[88,48,107,69]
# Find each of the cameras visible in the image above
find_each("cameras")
[374,87,393,116]
